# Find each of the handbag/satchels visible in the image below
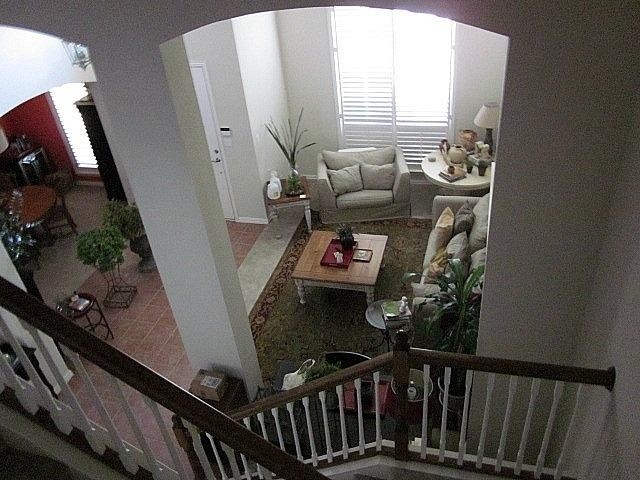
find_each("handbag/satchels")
[281,359,318,392]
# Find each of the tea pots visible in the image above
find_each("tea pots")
[448,144,467,164]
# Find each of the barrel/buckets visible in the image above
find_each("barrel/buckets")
[437,377,464,412]
[390,368,435,404]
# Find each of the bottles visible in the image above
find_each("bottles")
[407,380,419,401]
[448,166,455,174]
[457,129,478,150]
[439,139,450,154]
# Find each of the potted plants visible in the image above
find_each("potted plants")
[262,107,317,197]
[77,199,157,280]
[413,257,484,414]
[335,223,359,250]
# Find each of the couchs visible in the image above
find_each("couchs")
[412,194,488,328]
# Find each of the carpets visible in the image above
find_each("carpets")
[245,208,435,454]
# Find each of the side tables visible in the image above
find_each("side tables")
[366,299,409,355]
[264,176,312,239]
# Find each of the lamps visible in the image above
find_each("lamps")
[473,101,499,152]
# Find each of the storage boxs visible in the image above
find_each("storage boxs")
[188,369,227,401]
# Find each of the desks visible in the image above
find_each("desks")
[55,293,114,341]
[3,185,58,248]
[423,145,492,196]
[187,377,249,426]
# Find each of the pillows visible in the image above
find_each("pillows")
[326,162,395,195]
[423,199,475,287]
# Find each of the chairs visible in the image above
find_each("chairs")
[315,145,412,225]
[45,193,79,240]
